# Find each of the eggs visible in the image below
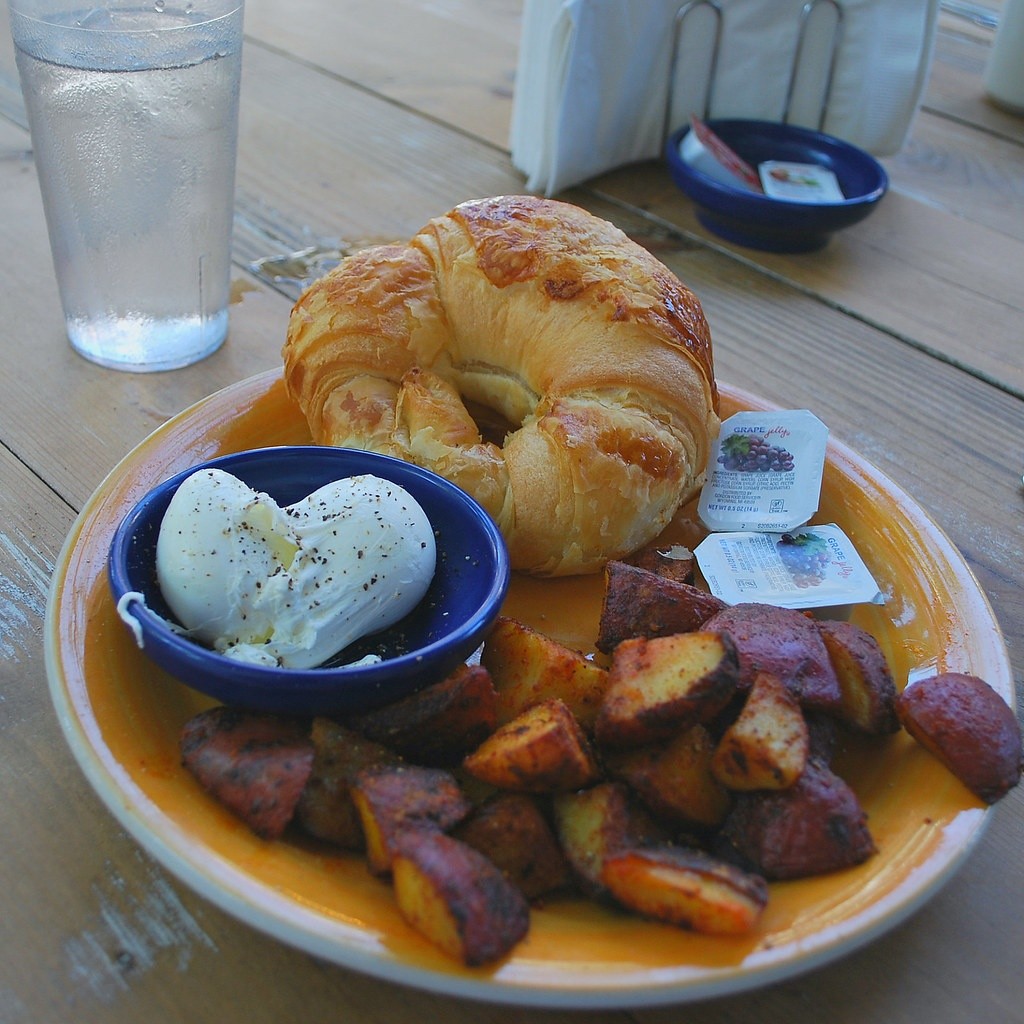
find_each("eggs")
[156,469,296,648]
[219,472,437,667]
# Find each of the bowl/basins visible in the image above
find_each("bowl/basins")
[107,444,511,714]
[666,118,888,253]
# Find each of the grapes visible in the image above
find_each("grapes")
[776,534,832,589]
[738,434,794,471]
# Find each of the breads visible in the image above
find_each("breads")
[282,194,725,577]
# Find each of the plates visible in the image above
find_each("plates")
[41,363,1016,1009]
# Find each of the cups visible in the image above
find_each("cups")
[7,0,245,373]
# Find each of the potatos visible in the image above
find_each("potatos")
[168,560,1024,966]
